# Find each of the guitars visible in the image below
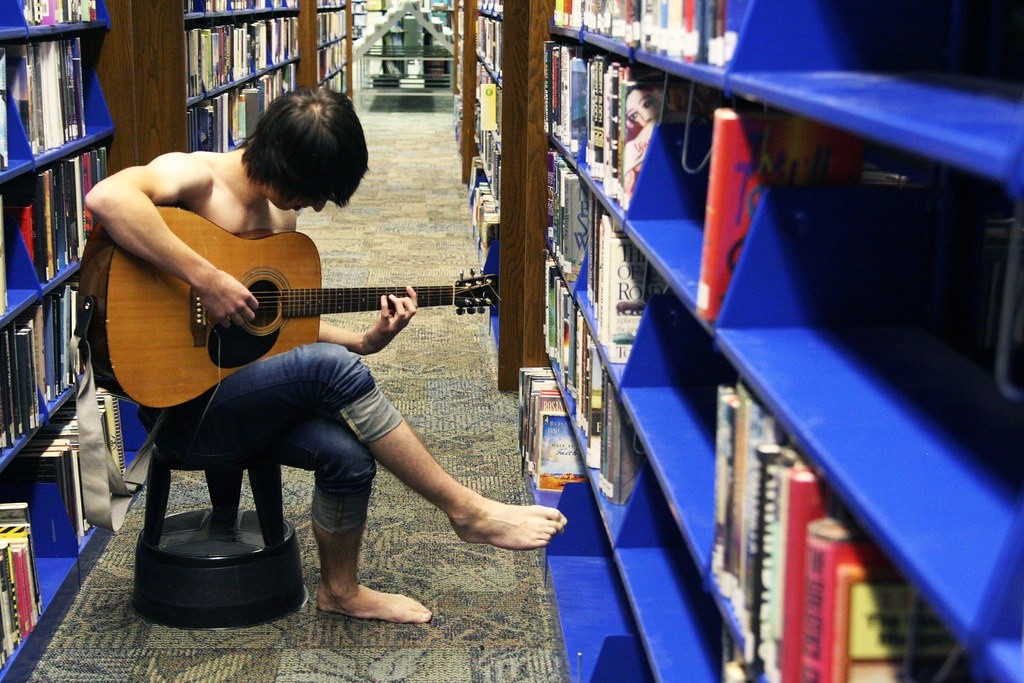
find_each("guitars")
[78,203,501,411]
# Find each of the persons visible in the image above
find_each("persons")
[71,84,572,626]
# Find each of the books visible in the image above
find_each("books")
[13,0,101,36]
[534,257,644,510]
[315,38,352,82]
[178,0,297,18]
[2,272,96,469]
[183,14,301,99]
[696,375,972,683]
[549,1,754,83]
[473,0,504,23]
[532,37,697,219]
[535,142,663,363]
[690,99,875,334]
[472,18,505,79]
[468,102,506,203]
[188,61,299,159]
[0,385,128,674]
[7,142,114,294]
[315,8,349,47]
[321,65,349,97]
[315,0,348,10]
[514,362,589,497]
[470,60,504,142]
[0,33,90,174]
[467,152,502,275]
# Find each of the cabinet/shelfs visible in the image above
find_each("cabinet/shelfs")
[0,0,371,683]
[451,2,1022,683]
